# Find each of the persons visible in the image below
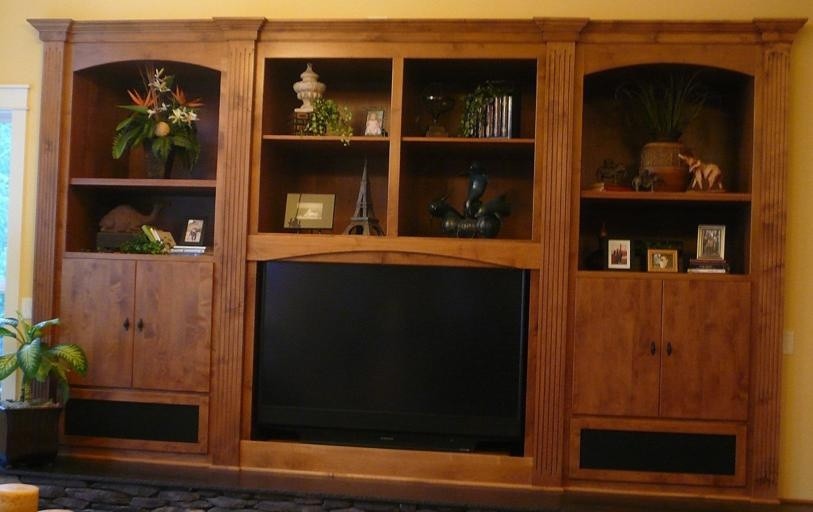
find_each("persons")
[364,110,381,136]
[611,229,721,268]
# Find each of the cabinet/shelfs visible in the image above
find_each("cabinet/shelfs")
[24,17,265,486]
[559,19,810,511]
[239,19,587,489]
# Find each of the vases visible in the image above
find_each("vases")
[141,138,174,180]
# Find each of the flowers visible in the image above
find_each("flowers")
[106,60,199,170]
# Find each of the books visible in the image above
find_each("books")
[141,224,208,256]
[467,91,518,139]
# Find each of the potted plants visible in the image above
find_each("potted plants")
[618,72,707,189]
[0,308,86,467]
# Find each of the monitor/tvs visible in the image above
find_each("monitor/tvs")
[248,263,531,460]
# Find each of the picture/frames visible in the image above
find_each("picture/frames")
[179,215,208,246]
[646,248,680,273]
[604,235,634,271]
[284,191,337,234]
[363,108,385,136]
[696,223,726,261]
